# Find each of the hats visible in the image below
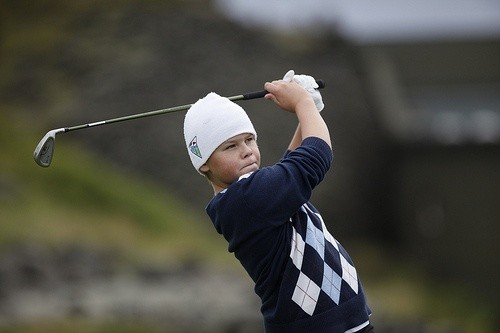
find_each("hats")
[183,93,257,178]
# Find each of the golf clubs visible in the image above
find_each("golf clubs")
[32,80,326,169]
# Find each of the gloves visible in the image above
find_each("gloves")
[283,70,325,114]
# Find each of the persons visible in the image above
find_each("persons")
[183,80,379,333]
